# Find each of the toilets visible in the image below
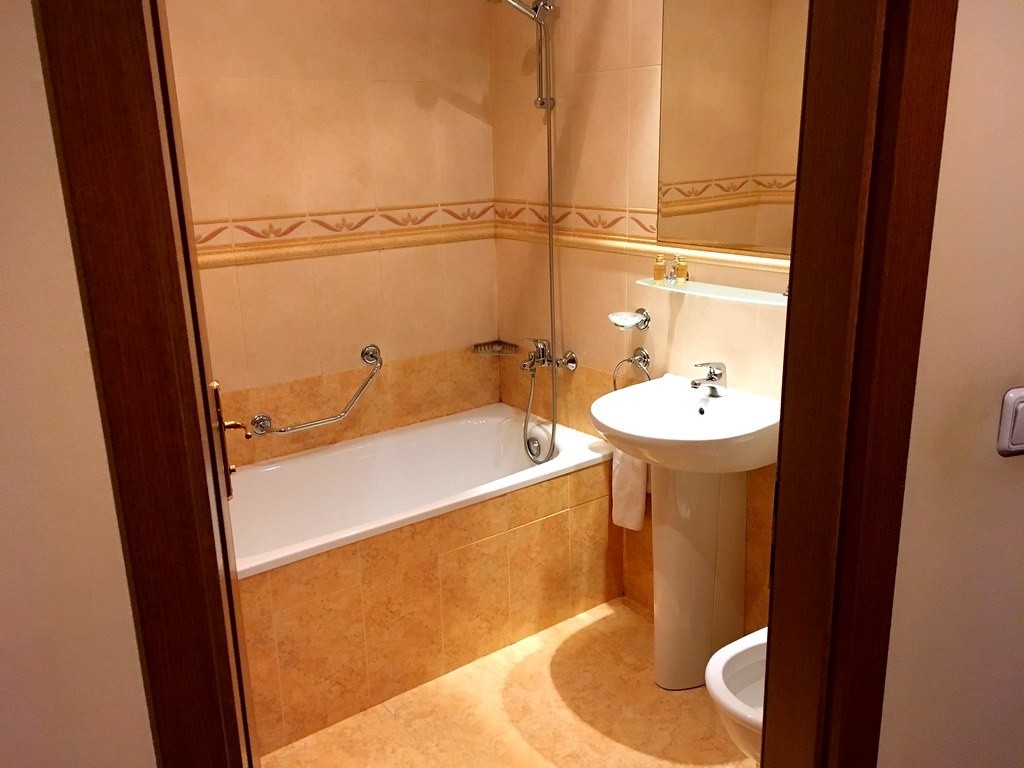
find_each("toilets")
[704,623,769,768]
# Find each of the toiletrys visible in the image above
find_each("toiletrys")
[675,257,688,287]
[653,254,667,283]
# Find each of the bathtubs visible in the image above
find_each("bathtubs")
[228,402,617,756]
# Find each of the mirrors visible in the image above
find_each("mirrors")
[656,1,810,253]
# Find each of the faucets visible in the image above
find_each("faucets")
[520,337,550,370]
[691,361,728,389]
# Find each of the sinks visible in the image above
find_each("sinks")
[588,373,781,473]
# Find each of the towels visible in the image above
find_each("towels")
[610,446,648,532]
